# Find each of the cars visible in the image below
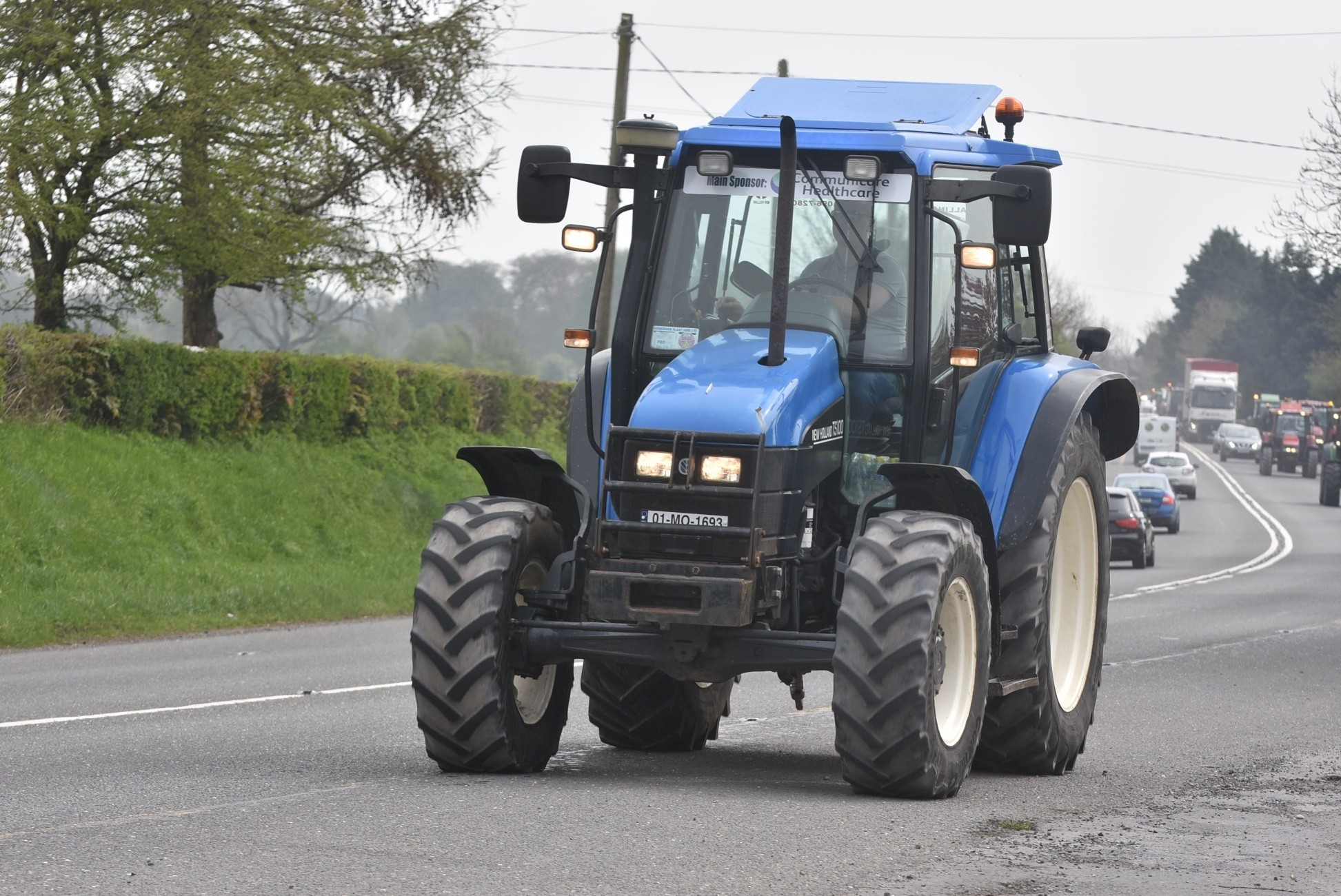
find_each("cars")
[1111,473,1188,534]
[1218,425,1263,464]
[1103,486,1155,571]
[1211,423,1247,453]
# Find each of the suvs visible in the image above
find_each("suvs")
[1139,451,1200,500]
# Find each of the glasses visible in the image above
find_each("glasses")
[831,209,871,222]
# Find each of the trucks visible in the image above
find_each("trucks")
[1138,383,1184,422]
[1176,358,1243,443]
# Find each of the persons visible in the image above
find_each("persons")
[715,200,909,366]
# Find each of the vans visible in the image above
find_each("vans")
[1133,415,1181,465]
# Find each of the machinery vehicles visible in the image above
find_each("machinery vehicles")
[411,75,1141,801]
[1246,392,1341,507]
